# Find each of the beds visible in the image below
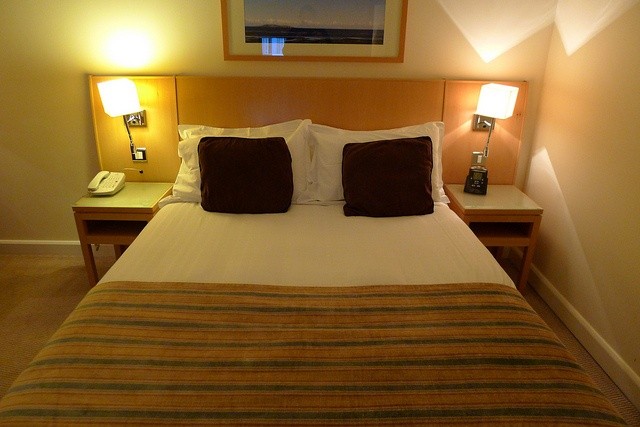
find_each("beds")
[0,73,630,424]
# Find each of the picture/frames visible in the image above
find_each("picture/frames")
[228,0,405,58]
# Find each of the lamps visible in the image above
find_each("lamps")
[467,82,519,158]
[96,77,143,153]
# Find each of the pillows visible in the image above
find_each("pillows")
[341,135,435,217]
[297,121,451,206]
[160,118,313,210]
[196,137,296,214]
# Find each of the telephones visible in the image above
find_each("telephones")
[87,170,125,197]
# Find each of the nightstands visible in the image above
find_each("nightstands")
[72,182,173,291]
[444,183,544,291]
